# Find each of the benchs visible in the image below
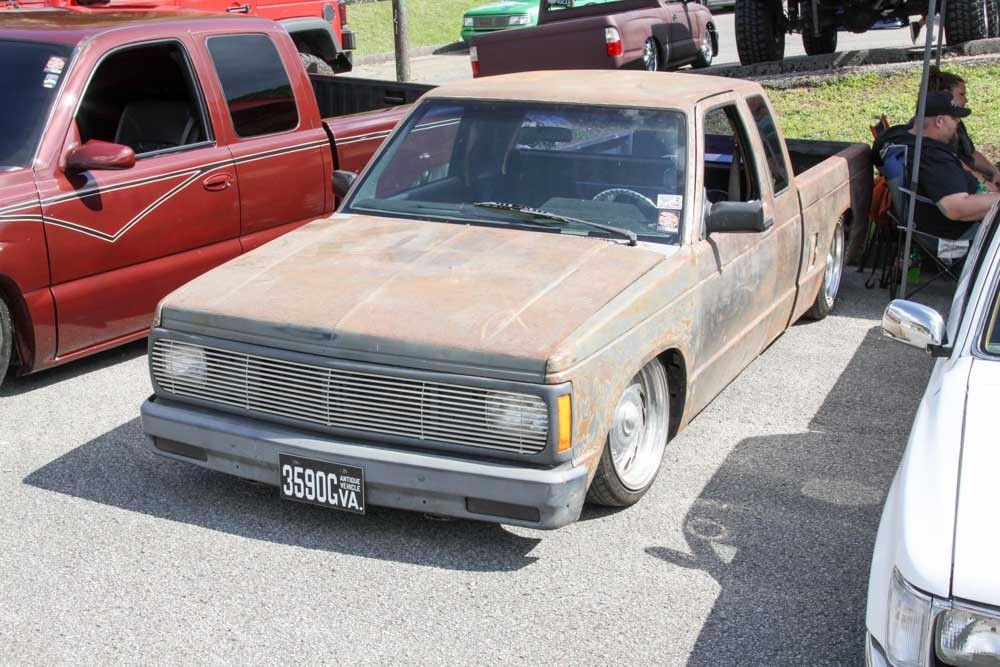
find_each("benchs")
[505,149,749,209]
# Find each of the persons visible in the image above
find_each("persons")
[873,91,1000,247]
[910,66,1000,195]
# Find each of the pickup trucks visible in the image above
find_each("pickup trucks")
[469,0,719,85]
[0,7,465,389]
[138,66,877,529]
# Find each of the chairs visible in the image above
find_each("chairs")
[113,95,201,153]
[464,174,535,206]
[857,116,984,301]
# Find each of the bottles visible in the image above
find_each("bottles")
[894,248,922,285]
[975,183,988,195]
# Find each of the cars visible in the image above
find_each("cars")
[856,186,1000,667]
[459,0,615,44]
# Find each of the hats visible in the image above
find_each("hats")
[917,90,972,117]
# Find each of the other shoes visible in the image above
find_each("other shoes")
[910,21,921,45]
